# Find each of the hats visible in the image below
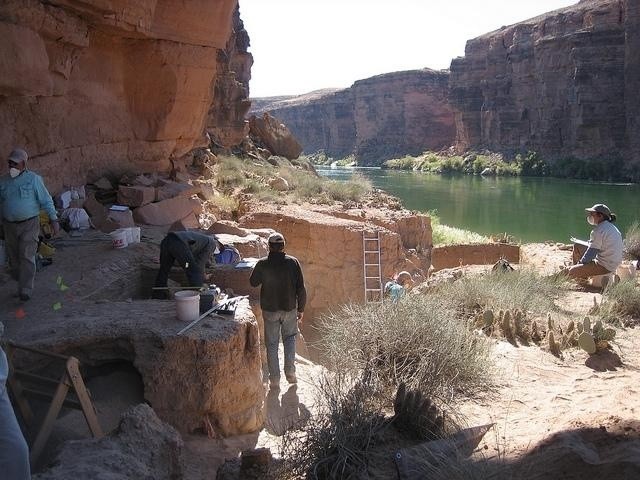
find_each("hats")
[7,149,28,164]
[398,271,415,286]
[268,232,285,243]
[585,203,612,217]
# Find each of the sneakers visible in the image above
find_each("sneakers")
[284,369,298,384]
[12,291,32,301]
[269,376,281,389]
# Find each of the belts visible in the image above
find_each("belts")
[7,215,37,224]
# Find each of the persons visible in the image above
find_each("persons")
[0,324,31,480]
[154,230,224,292]
[1,148,59,301]
[384,270,412,302]
[540,203,623,286]
[249,232,306,383]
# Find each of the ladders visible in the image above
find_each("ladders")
[362,231,384,306]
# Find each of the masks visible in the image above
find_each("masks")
[214,246,220,255]
[587,215,595,226]
[9,168,21,178]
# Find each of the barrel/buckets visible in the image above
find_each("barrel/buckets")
[175,290,200,321]
[132,226,140,243]
[116,227,134,244]
[110,230,128,249]
